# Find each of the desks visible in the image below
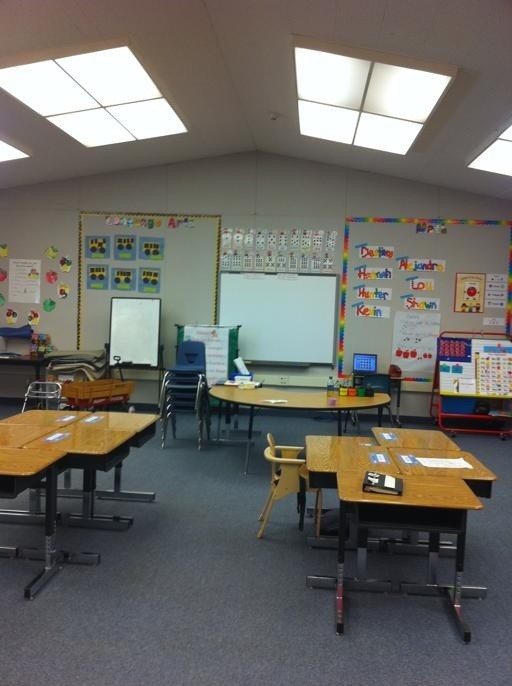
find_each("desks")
[0,353,55,409]
[350,374,406,426]
[206,382,393,480]
[1,408,162,603]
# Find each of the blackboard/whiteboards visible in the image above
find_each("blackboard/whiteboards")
[217,271,341,368]
[109,297,161,369]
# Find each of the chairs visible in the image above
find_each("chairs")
[158,340,215,455]
[349,374,393,425]
[256,431,324,539]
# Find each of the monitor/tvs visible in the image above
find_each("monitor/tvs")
[354,353,377,376]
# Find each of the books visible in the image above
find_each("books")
[214,379,261,387]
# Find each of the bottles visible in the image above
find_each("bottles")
[326,374,335,400]
